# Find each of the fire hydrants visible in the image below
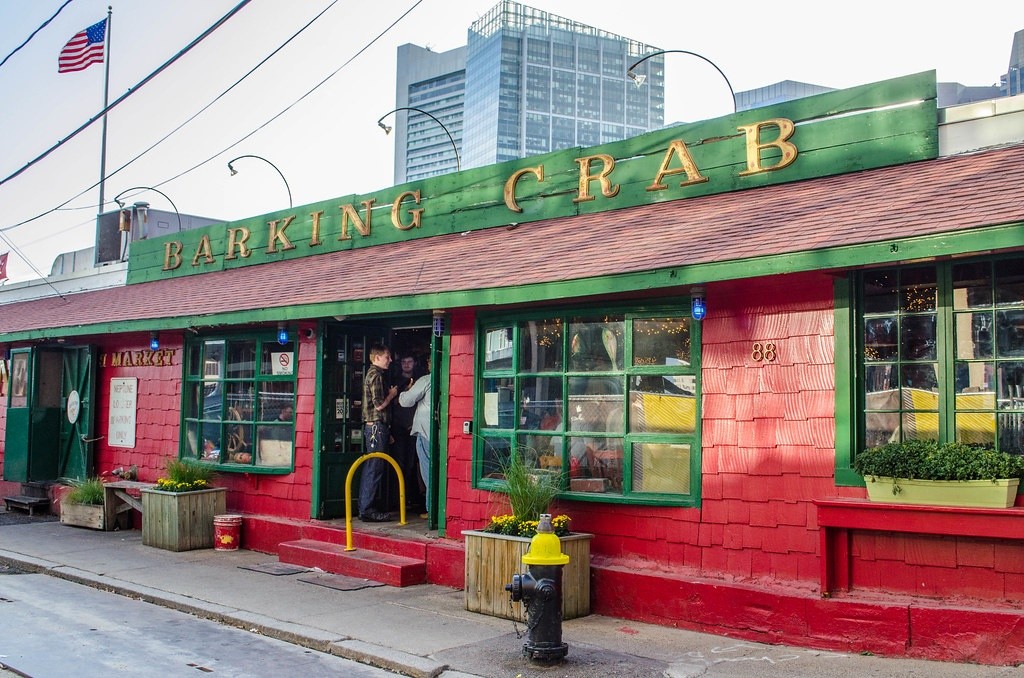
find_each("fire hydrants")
[505,513,570,664]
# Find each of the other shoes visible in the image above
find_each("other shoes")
[420,514,428,520]
[358,512,391,522]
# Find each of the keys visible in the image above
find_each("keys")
[370,425,378,448]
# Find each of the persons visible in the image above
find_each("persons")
[387,348,432,520]
[357,344,399,523]
[269,404,295,441]
[638,375,673,395]
[540,402,601,483]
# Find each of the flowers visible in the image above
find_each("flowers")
[152,459,219,492]
[466,433,582,537]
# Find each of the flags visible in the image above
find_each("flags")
[58,17,106,73]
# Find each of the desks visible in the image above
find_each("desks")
[102,480,159,531]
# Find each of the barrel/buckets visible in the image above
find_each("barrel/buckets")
[213,514,242,551]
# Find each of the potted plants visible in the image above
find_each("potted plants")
[55,476,104,529]
[850,438,1024,508]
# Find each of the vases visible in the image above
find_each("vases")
[140,486,228,549]
[462,529,593,622]
